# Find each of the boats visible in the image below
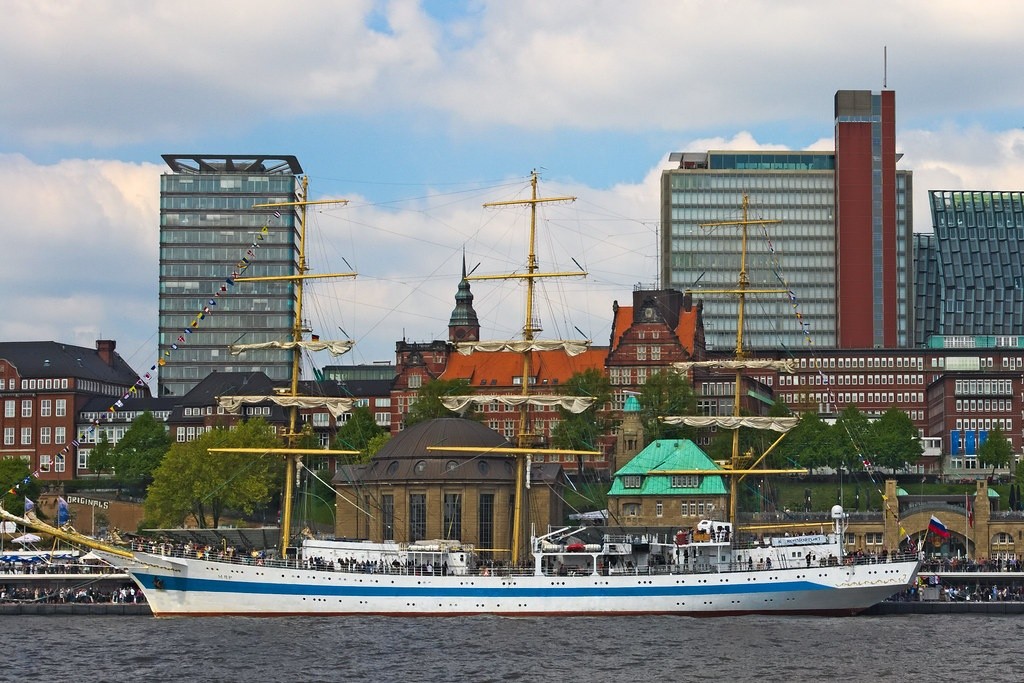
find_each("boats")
[0,160,1024,620]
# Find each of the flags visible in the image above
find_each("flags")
[0,204,287,503]
[759,226,976,565]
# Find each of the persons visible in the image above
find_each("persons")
[13,477,1022,619]
